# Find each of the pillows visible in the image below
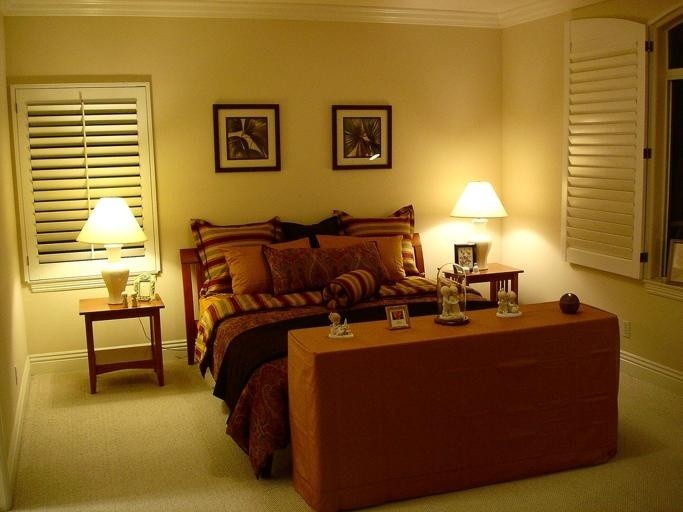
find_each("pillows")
[279,217,339,248]
[189,215,278,297]
[220,236,312,295]
[316,234,407,284]
[260,240,390,296]
[322,266,379,307]
[330,204,421,278]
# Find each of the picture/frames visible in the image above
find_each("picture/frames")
[211,102,281,172]
[454,243,475,271]
[663,238,682,286]
[385,305,411,330]
[331,103,392,170]
[132,272,156,302]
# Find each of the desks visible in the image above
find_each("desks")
[78,293,165,394]
[438,264,524,311]
[285,300,620,512]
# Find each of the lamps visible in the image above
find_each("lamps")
[76,197,149,306]
[449,181,509,271]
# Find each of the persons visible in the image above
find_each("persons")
[130,292,138,306]
[497,288,507,313]
[449,284,461,315]
[440,285,448,315]
[328,313,341,328]
[120,291,127,307]
[506,291,519,313]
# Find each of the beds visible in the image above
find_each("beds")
[178,232,500,482]
